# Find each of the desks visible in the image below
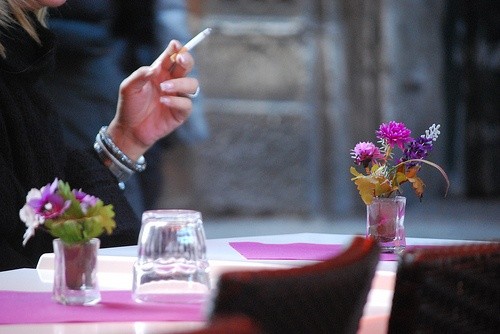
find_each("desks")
[0,233,500,334]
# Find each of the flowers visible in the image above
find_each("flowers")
[19,177,116,246]
[350,120,450,205]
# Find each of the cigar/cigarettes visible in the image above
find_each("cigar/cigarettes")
[171,28,212,63]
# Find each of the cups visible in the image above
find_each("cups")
[132,208,211,304]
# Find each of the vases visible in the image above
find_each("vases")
[54,238,101,306]
[365,196,407,261]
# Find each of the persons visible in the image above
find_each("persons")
[0,0,213,273]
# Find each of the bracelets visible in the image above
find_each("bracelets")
[96,132,134,177]
[98,124,146,172]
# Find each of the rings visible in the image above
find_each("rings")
[187,87,200,98]
